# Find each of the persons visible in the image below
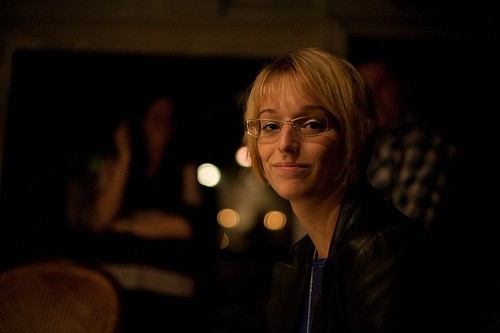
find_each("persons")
[41,116,200,311]
[348,53,454,229]
[209,48,461,332]
[123,97,202,216]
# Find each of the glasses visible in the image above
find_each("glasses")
[245,114,338,139]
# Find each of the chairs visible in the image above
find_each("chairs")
[0,263,120,333]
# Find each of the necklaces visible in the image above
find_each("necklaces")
[305,247,319,332]
